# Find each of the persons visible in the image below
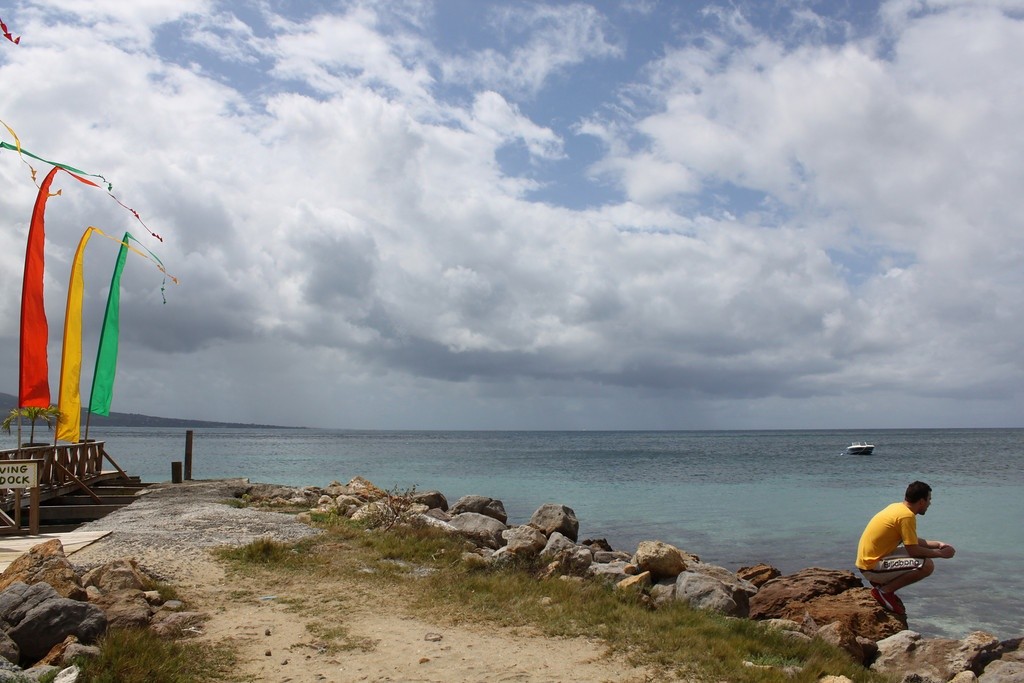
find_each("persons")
[854,481,957,613]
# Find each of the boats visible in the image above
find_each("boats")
[846,441,874,454]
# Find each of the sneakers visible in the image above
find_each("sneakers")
[872,584,904,614]
[870,581,899,601]
